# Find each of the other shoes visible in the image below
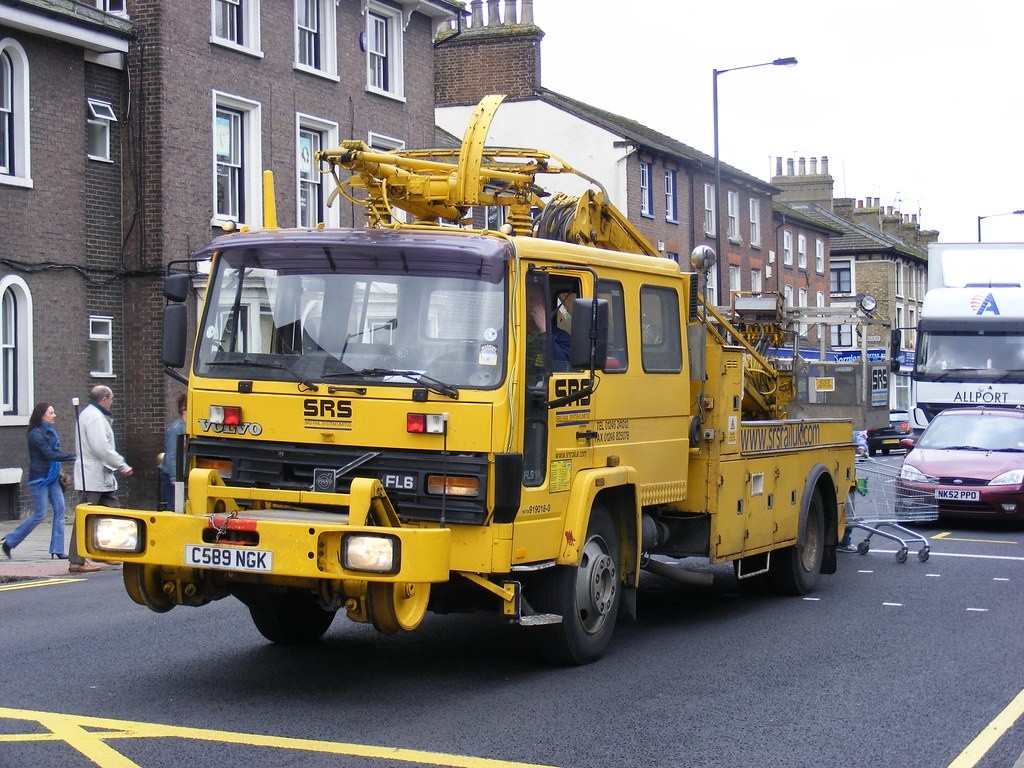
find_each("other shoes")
[92,558,122,565]
[69,563,101,572]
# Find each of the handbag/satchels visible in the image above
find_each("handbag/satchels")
[58,470,72,493]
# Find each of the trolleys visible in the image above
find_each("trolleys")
[845,445,939,563]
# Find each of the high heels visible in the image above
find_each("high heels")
[1,537,11,559]
[52,553,69,559]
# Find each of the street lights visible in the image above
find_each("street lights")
[978,210,1023,241]
[712,56,798,308]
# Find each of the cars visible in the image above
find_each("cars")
[895,405,1024,527]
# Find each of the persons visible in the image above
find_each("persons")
[158,394,189,513]
[1,403,75,559]
[837,429,870,552]
[68,385,133,572]
[484,282,571,373]
[926,342,958,368]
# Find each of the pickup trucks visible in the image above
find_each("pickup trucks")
[867,408,915,457]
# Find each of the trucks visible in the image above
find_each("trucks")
[890,240,1024,448]
[76,89,856,666]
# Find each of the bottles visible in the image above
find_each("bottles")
[987,358,992,370]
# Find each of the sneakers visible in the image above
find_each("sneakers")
[836,543,858,553]
